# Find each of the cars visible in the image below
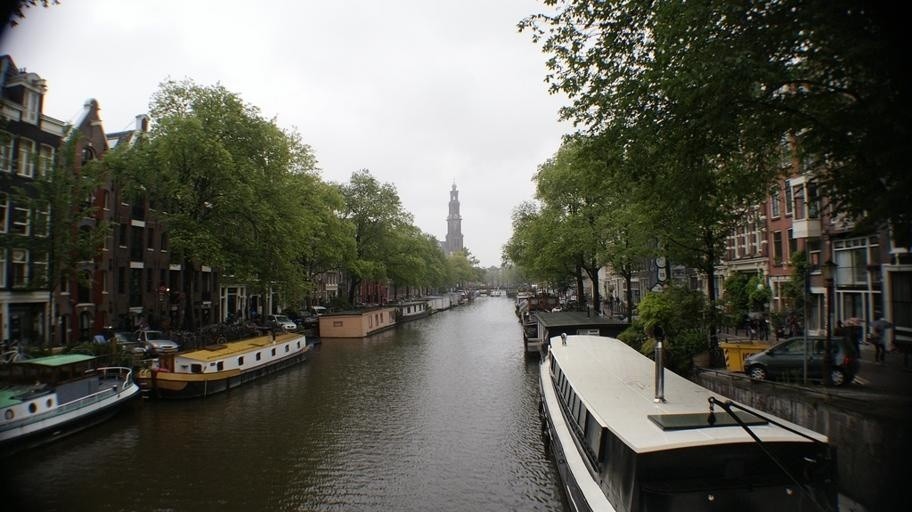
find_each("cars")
[265,313,297,332]
[743,335,862,388]
[107,327,179,357]
[302,305,330,325]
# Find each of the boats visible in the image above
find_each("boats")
[134,332,322,399]
[490,290,502,298]
[0,354,141,443]
[398,287,487,324]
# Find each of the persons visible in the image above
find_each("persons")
[599,294,627,313]
[743,318,806,342]
[813,319,862,359]
[871,327,888,361]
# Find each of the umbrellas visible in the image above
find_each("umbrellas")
[843,316,864,327]
[867,318,893,329]
